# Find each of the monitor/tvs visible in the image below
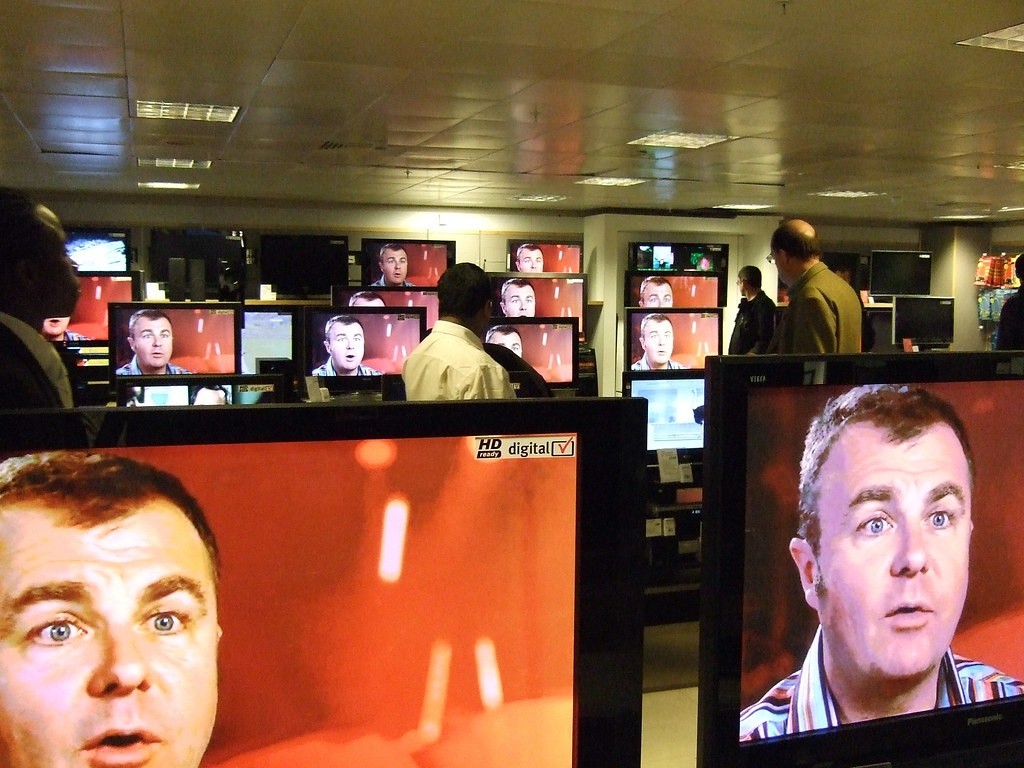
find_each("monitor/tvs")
[0,227,1023,768]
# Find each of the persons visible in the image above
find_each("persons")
[312,314,382,376]
[349,291,386,306]
[767,219,862,353]
[739,382,1024,743]
[996,254,1024,350]
[639,276,673,307]
[486,325,522,359]
[833,266,876,352]
[632,313,687,370]
[728,265,776,356]
[189,385,229,405]
[371,243,412,286]
[499,279,535,317]
[516,244,544,273]
[0,451,223,768]
[0,186,82,408]
[402,262,517,401]
[116,309,192,375]
[42,316,90,341]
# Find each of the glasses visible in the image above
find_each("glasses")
[766,250,777,264]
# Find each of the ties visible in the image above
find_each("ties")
[54,359,74,409]
